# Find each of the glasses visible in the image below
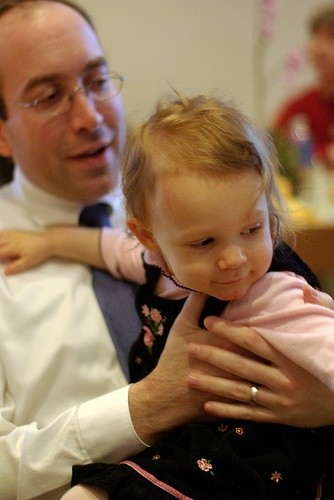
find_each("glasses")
[3,73,124,114]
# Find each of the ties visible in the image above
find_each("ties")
[80,201,142,382]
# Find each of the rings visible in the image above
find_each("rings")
[249,383,258,408]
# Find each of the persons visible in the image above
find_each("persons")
[1,83,334,500]
[269,7,334,300]
[1,1,334,500]
